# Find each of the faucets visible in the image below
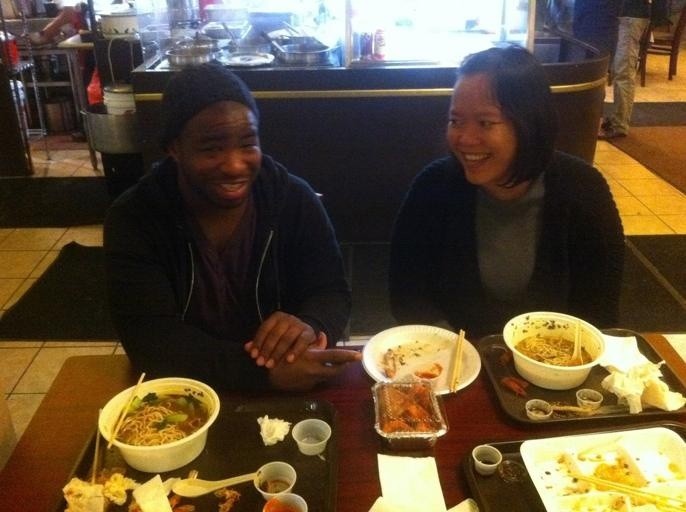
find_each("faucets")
[344,0,352,68]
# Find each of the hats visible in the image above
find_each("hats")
[154,58,262,154]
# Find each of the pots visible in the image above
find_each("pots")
[99,13,138,39]
[166,38,223,66]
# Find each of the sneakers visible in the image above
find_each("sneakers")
[596,118,630,142]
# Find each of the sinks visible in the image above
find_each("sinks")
[352,60,441,68]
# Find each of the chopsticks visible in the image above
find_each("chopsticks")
[568,469,682,508]
[87,407,102,489]
[448,328,467,400]
[107,369,149,449]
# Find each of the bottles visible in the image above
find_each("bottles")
[362,31,387,60]
[44,1,58,17]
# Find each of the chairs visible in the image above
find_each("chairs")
[608,18,651,86]
[638,5,686,79]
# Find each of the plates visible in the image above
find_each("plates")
[359,324,483,396]
[521,427,686,511]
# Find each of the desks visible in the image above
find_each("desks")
[9,59,50,173]
[58,34,95,132]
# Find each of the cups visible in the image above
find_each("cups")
[575,390,603,409]
[471,443,504,476]
[293,419,333,454]
[497,461,528,491]
[523,397,554,419]
[254,461,307,512]
[2,37,18,65]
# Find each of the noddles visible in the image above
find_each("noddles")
[515,335,593,367]
[114,401,201,446]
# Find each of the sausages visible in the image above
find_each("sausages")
[384,350,396,378]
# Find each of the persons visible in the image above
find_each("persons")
[591,0,652,141]
[389,46,626,342]
[102,60,364,395]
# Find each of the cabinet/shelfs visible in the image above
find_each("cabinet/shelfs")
[18,47,81,126]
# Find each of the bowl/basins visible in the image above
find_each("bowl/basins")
[503,311,611,391]
[271,44,333,65]
[99,377,223,475]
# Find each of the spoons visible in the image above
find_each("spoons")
[171,468,264,499]
